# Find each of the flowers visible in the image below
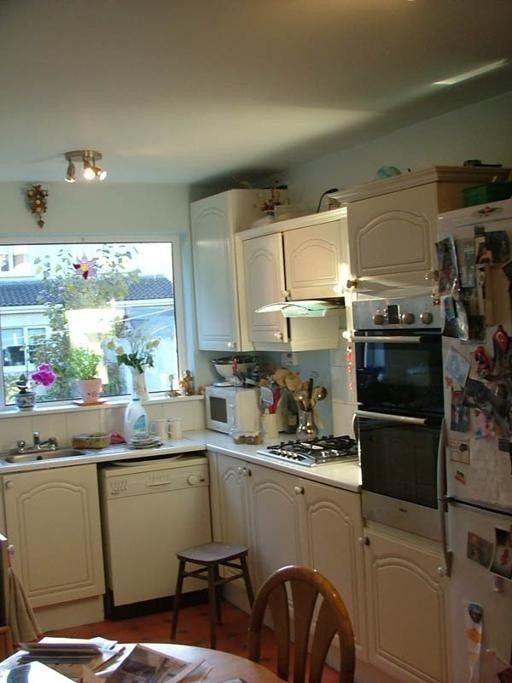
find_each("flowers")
[108,326,161,374]
[15,363,55,393]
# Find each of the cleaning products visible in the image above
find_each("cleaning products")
[124,397,149,445]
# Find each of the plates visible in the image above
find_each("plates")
[130,436,163,449]
[70,399,107,405]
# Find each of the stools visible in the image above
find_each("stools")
[170,541,253,650]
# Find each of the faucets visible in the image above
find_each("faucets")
[32,432,40,449]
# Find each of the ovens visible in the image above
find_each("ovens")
[351,296,449,541]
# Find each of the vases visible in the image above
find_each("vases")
[15,393,35,411]
[125,365,149,400]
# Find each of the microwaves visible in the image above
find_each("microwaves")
[203,385,290,435]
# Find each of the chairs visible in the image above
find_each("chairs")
[250,565,355,683]
[0,533,13,657]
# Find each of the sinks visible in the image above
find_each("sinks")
[3,449,91,465]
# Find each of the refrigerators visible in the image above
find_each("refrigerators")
[435,323,512,682]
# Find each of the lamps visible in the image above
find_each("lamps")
[64,150,107,183]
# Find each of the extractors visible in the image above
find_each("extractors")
[254,299,345,320]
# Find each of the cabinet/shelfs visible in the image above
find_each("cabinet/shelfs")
[235,208,351,352]
[189,189,286,353]
[184,428,362,683]
[360,491,457,683]
[328,163,512,517]
[0,448,107,632]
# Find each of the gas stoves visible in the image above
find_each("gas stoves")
[256,434,358,468]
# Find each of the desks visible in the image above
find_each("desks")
[0,642,283,682]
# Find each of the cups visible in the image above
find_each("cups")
[166,417,183,440]
[150,419,168,440]
[261,413,281,439]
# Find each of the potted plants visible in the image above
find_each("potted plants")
[67,347,102,402]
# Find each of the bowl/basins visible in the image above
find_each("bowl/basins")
[209,355,256,382]
